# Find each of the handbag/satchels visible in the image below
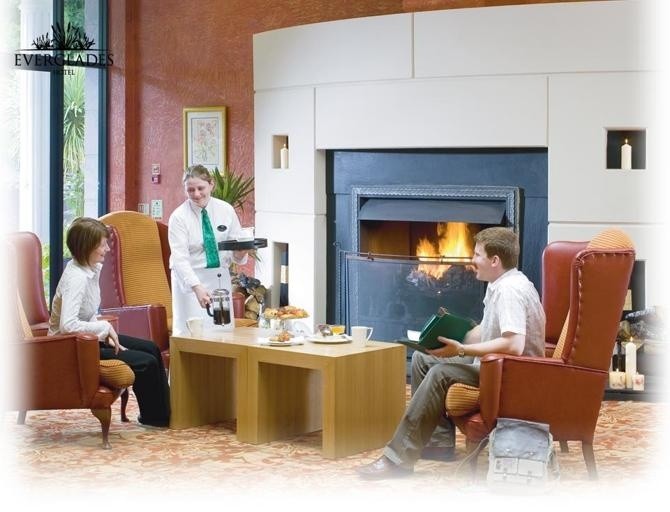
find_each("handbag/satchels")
[453,416,562,491]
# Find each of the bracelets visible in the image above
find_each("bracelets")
[459,342,466,356]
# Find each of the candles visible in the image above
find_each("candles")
[608,367,625,389]
[625,335,636,388]
[621,138,632,169]
[280,144,288,169]
[633,371,645,392]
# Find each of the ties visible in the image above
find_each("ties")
[201,209,221,269]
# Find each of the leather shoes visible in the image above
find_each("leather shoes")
[357,456,414,478]
[420,446,454,462]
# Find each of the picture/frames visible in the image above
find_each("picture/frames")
[183,106,228,179]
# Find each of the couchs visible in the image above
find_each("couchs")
[95,209,175,369]
[14,230,130,450]
[447,226,636,483]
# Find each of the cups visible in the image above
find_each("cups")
[205,287,231,328]
[269,319,281,334]
[236,224,254,240]
[351,325,374,350]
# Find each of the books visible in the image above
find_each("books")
[395,311,477,354]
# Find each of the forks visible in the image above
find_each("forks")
[341,335,351,342]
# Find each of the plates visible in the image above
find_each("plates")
[217,237,269,251]
[267,337,292,348]
[306,334,352,345]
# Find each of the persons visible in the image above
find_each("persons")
[358,227,546,481]
[168,164,251,335]
[48,217,172,428]
